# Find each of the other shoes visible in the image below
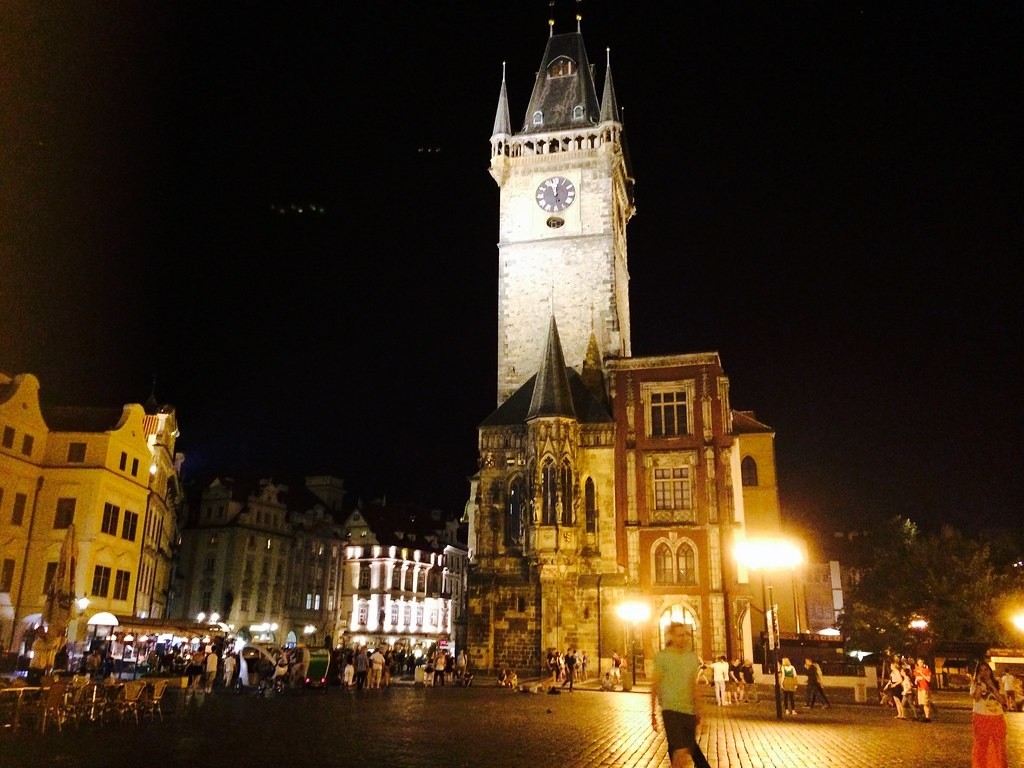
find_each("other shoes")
[894,716,906,719]
[786,709,790,715]
[792,710,798,715]
[911,718,919,721]
[922,718,931,723]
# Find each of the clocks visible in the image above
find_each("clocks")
[535,175,575,212]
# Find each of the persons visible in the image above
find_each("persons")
[497,668,518,688]
[774,658,799,714]
[883,656,931,723]
[710,655,762,706]
[27,626,46,678]
[803,658,832,709]
[53,646,116,680]
[996,667,1024,712]
[651,620,700,768]
[610,649,627,683]
[599,673,615,692]
[124,642,474,694]
[696,656,709,685]
[970,662,1008,768]
[546,647,589,692]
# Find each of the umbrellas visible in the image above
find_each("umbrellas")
[40,523,81,676]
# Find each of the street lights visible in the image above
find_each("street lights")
[734,538,802,718]
[617,603,650,685]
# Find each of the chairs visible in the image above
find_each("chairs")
[24,680,167,738]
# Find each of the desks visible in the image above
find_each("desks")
[1,685,80,733]
